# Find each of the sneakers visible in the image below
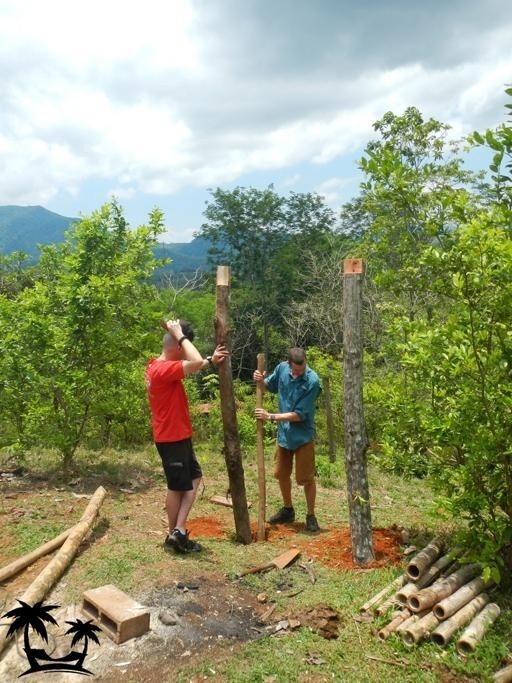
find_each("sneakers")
[306,515,318,532]
[270,507,295,522]
[165,529,201,554]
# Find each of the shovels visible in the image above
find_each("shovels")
[235,548,302,579]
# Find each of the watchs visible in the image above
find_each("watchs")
[271,413,275,424]
[207,355,214,370]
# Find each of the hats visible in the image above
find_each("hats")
[160,320,193,343]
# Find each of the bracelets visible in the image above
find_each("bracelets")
[177,335,189,349]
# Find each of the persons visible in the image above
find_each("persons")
[144,320,230,554]
[252,347,322,531]
